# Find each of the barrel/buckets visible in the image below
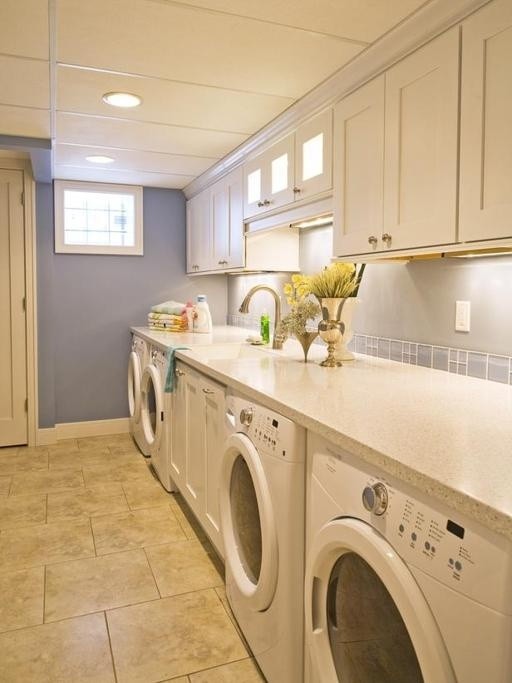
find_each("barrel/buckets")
[261,307,270,343]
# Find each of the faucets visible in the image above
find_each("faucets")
[238,284,283,349]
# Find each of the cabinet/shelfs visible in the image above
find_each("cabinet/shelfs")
[166,358,227,566]
[334,1,512,264]
[185,165,299,276]
[240,83,332,236]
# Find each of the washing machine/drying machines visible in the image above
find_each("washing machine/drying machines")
[222,387,305,683]
[303,428,512,683]
[126,330,174,491]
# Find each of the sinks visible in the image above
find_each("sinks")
[188,342,265,360]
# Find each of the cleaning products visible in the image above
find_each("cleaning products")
[195,295,211,333]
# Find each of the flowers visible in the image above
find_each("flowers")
[274,299,322,337]
[317,297,347,368]
[283,261,364,306]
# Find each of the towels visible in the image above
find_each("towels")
[161,345,191,392]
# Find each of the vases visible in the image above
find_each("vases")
[296,332,319,363]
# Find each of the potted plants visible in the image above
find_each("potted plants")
[325,258,366,361]
